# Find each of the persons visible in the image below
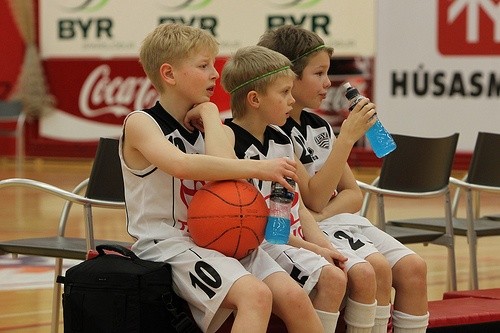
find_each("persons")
[256,26,430,333]
[220,45,348,333]
[118,23,325,333]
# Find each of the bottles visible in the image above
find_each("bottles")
[344,82,397,158]
[265,156,295,244]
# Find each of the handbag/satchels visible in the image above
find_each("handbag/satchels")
[56,244,204,333]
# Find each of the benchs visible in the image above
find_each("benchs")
[386,288,499,333]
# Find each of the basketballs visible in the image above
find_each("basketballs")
[187,179,269,260]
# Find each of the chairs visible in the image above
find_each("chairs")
[0,138,154,333]
[388,130,500,291]
[355,131,461,292]
